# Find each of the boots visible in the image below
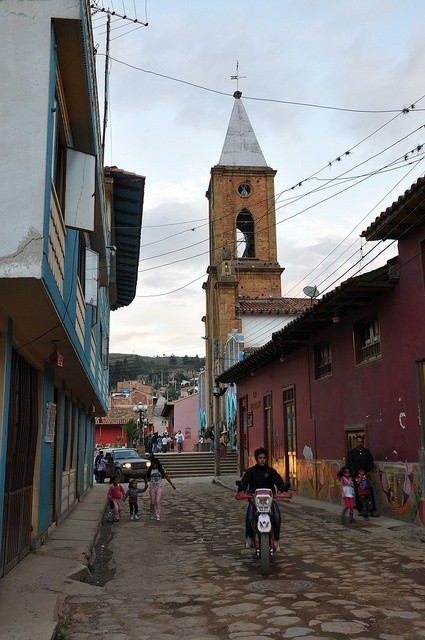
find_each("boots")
[341,511,346,525]
[349,512,357,524]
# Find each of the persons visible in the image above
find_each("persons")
[192,434,204,451]
[107,476,126,522]
[376,462,414,509]
[208,430,214,452]
[305,460,314,489]
[296,460,302,490]
[104,452,113,463]
[345,435,380,518]
[149,430,174,453]
[355,467,373,522]
[218,441,227,459]
[327,462,343,505]
[175,430,185,453]
[94,451,104,483]
[236,447,288,553]
[144,458,177,522]
[318,459,325,492]
[340,469,356,525]
[121,479,149,521]
[96,454,109,484]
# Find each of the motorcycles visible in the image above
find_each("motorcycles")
[233,489,292,575]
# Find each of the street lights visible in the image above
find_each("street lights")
[133,401,148,444]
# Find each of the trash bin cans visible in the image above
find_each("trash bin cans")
[194,443,199,452]
[199,443,205,452]
[205,443,211,452]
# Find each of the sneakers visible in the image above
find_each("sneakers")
[245,536,252,548]
[129,515,134,520]
[156,516,161,521]
[134,514,139,520]
[273,540,281,553]
[150,515,154,520]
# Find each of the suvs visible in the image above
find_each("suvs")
[95,449,151,482]
